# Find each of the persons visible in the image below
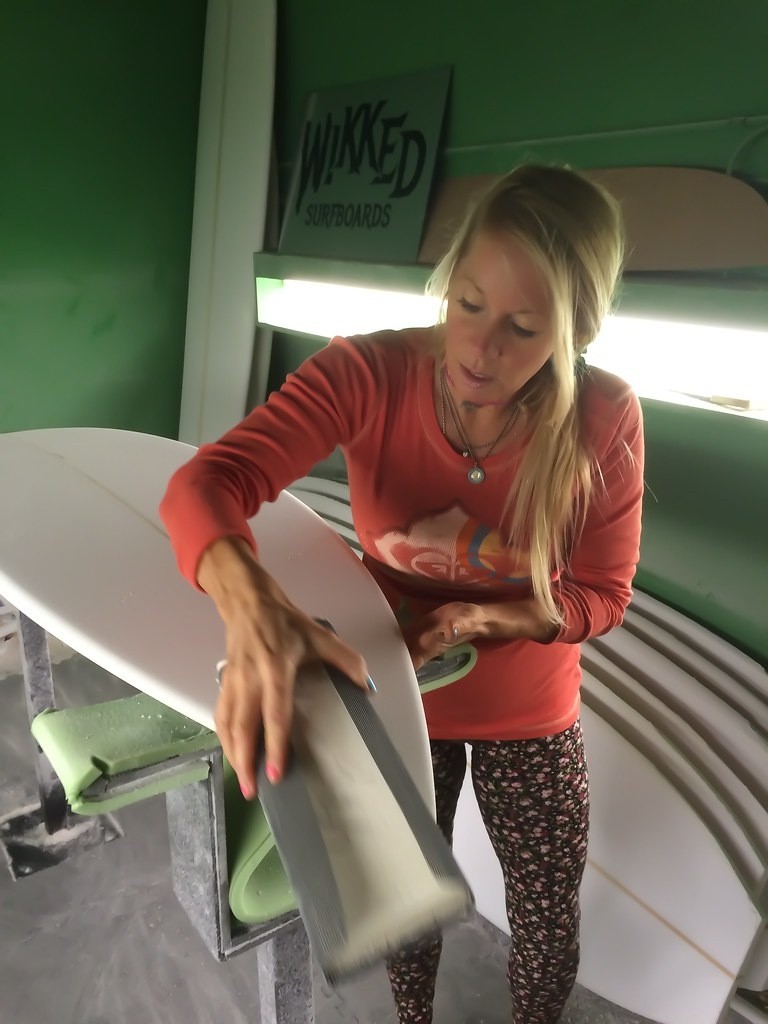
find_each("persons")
[161,163,643,1024]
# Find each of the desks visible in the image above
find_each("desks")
[0,427,437,1024]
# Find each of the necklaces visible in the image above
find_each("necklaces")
[437,366,520,486]
